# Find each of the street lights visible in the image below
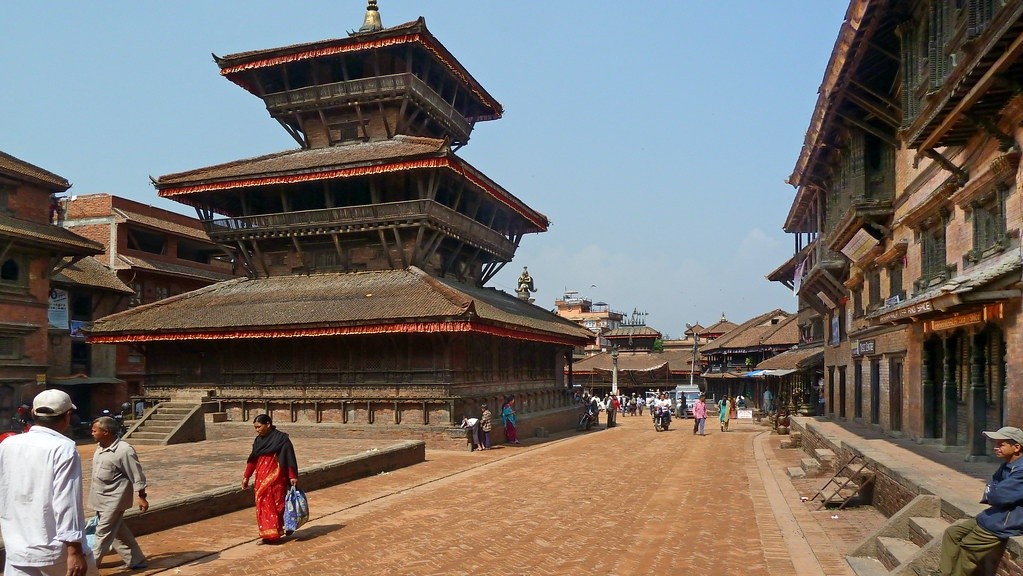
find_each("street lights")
[611,343,621,397]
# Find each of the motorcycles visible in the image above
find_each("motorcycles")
[651,406,672,432]
[577,397,599,430]
[102,409,126,438]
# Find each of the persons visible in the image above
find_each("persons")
[763,386,773,414]
[0,388,103,576]
[501,395,519,444]
[930,424,1023,575]
[479,402,493,450]
[458,412,486,450]
[242,414,300,546]
[574,388,744,434]
[87,416,151,571]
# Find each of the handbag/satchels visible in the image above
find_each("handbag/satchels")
[82,514,112,557]
[284,483,309,535]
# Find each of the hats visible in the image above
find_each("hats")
[32,389,77,416]
[981,427,1023,449]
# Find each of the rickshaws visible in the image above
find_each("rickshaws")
[627,403,637,416]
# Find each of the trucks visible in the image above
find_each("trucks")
[665,384,701,418]
